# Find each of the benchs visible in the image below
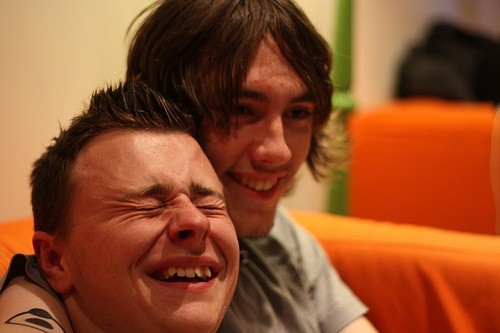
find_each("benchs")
[0,209,499,333]
[349,98,500,235]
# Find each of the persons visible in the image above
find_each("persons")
[0,0,378,333]
[30,76,240,333]
